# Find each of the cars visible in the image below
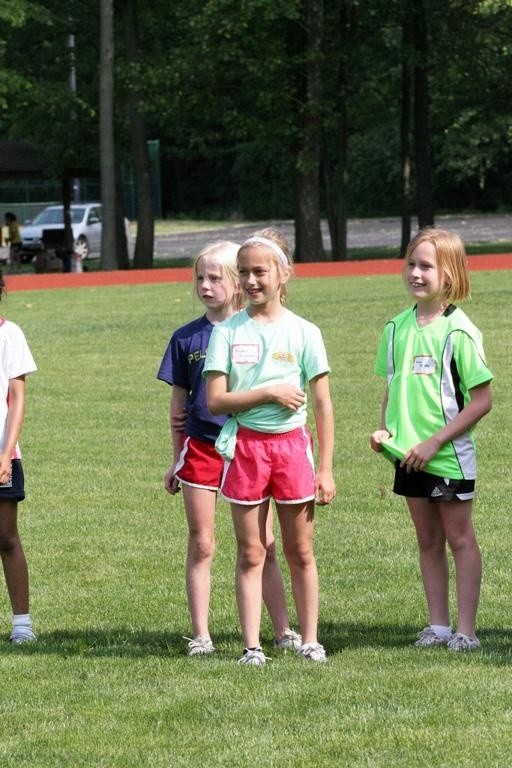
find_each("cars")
[16,202,131,265]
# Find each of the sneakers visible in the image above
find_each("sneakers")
[445,636,481,653]
[189,637,212,656]
[11,627,37,646]
[237,651,265,668]
[416,629,451,646]
[297,644,328,663]
[273,635,302,650]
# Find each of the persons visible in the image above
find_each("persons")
[368,228,494,653]
[4,211,23,274]
[0,267,39,649]
[202,227,337,668]
[156,240,304,656]
